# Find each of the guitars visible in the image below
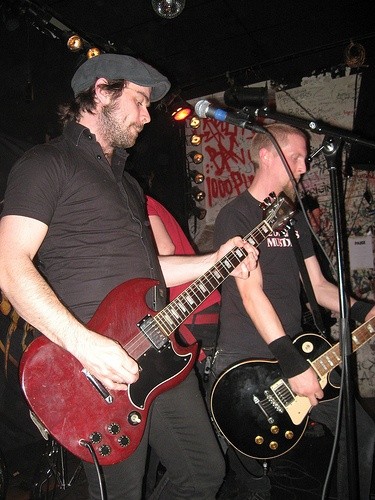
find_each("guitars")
[211,315,375,460]
[18,190,298,466]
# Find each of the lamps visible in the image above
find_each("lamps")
[47,0,207,221]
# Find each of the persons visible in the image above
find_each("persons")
[209,124,375,500]
[0,54,259,500]
[142,196,221,500]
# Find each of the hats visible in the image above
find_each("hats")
[71,54,170,103]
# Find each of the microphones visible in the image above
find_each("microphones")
[195,100,267,134]
